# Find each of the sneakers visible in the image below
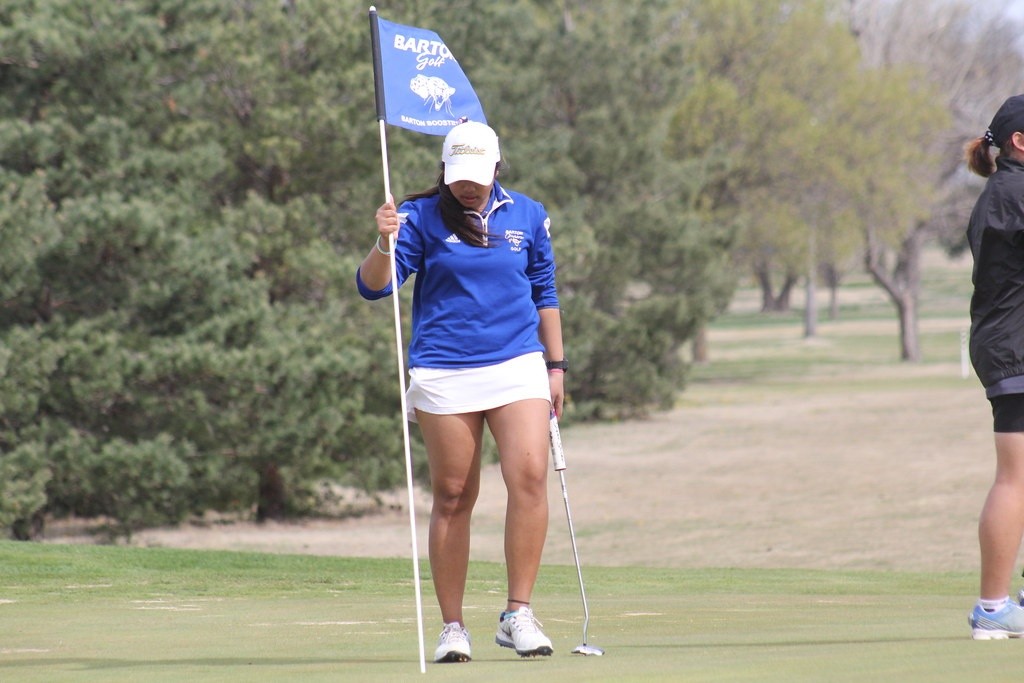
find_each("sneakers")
[1017,585,1024,606]
[434,621,471,662]
[968,599,1024,640]
[495,606,554,657]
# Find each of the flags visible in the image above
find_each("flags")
[377,16,487,136]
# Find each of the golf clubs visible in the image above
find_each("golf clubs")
[550,405,604,656]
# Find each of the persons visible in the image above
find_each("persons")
[967,93,1024,641]
[355,116,565,660]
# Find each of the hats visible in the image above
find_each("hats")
[441,120,500,187]
[988,94,1024,149]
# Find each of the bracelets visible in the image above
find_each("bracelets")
[547,369,564,374]
[377,235,397,255]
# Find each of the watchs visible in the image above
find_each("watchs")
[546,358,569,373]
